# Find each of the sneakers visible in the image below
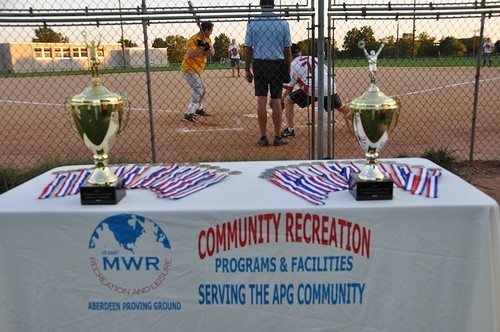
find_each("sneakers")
[257,137,269,147]
[273,136,289,145]
[281,128,296,138]
[196,108,207,116]
[183,113,199,124]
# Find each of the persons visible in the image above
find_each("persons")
[281,43,351,139]
[482,37,495,68]
[84,33,102,78]
[245,0,292,147]
[362,42,385,87]
[228,38,240,77]
[181,22,215,123]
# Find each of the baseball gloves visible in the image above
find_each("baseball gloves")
[233,51,237,56]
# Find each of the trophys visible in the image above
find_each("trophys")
[65,33,131,205]
[344,42,401,202]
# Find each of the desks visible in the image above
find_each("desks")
[0,157,500,332]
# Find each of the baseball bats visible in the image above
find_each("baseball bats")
[188,1,207,43]
